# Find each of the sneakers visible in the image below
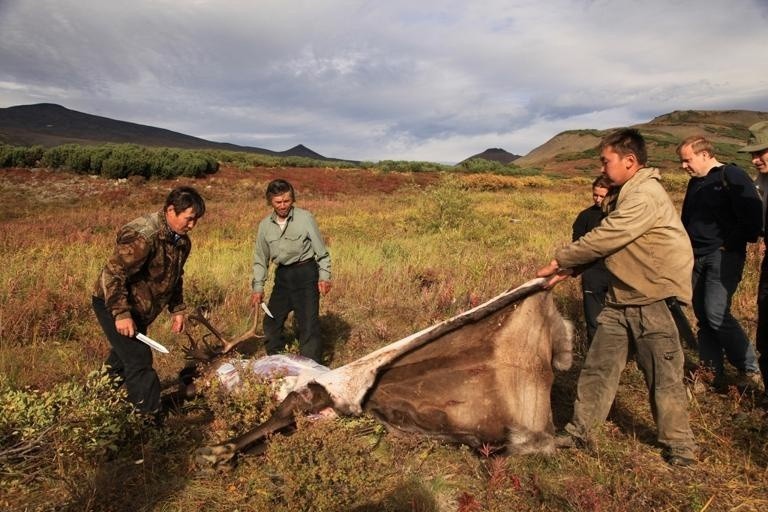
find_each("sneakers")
[692,374,720,394]
[739,374,763,395]
[553,430,580,448]
[668,456,697,466]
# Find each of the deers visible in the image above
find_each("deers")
[181,277,574,476]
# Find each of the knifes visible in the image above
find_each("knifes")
[128,330,171,353]
[258,302,274,319]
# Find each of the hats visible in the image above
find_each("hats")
[735,120,767,153]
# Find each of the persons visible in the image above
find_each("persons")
[91,186,206,416]
[250,178,333,363]
[571,176,614,351]
[536,126,698,470]
[736,119,768,409]
[675,134,764,391]
